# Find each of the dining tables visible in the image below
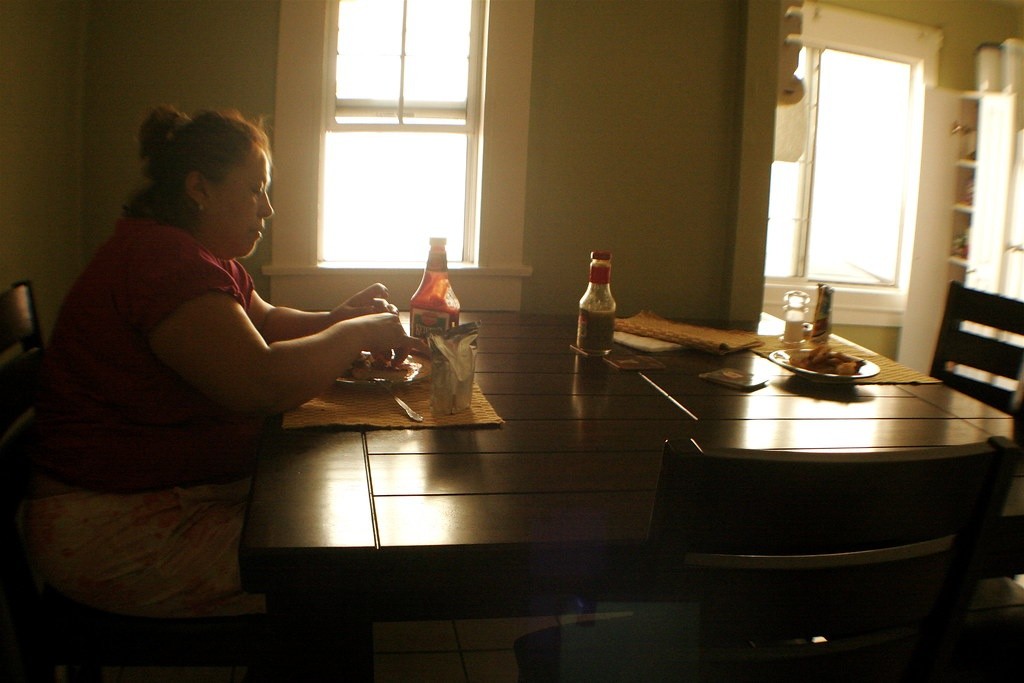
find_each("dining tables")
[238,309,1023,683]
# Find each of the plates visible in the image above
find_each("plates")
[333,348,436,390]
[769,348,881,382]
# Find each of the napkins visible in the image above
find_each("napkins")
[612,331,688,353]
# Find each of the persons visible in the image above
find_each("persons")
[17,105,433,620]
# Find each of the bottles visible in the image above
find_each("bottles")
[409,236,461,353]
[577,248,616,354]
[782,289,809,342]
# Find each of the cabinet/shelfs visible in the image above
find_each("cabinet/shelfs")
[896,87,1017,386]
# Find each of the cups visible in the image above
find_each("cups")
[429,350,476,413]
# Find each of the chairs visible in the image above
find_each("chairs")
[513,436,1022,683]
[0,279,375,683]
[918,279,1024,584]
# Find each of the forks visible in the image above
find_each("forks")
[372,377,423,422]
